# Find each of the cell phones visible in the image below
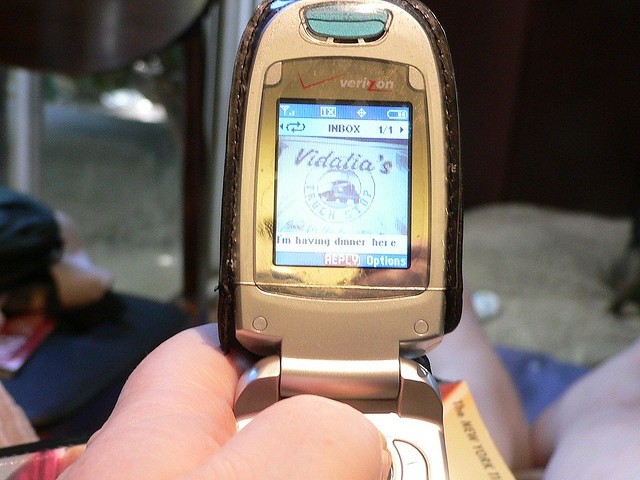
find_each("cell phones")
[218,0,463,479]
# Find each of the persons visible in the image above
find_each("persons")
[57,291,638,480]
